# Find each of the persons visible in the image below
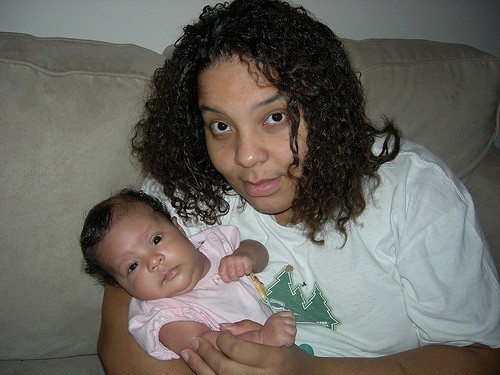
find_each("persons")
[76,189,297,360]
[95,0,500,375]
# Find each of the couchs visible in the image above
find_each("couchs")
[0,32,500,375]
